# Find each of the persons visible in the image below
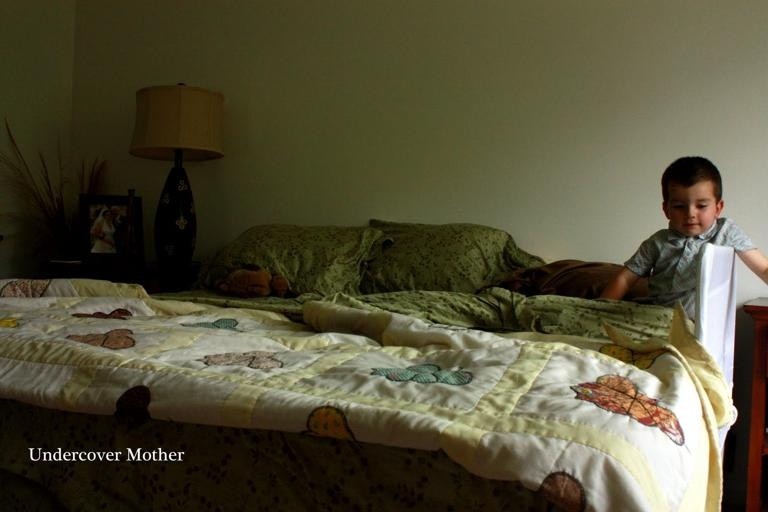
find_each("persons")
[597,156,768,324]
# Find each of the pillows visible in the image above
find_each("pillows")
[211,217,546,300]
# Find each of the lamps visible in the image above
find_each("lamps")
[129,83,225,276]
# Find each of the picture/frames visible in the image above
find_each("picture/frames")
[77,193,145,269]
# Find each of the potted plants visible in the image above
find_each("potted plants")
[1,116,112,278]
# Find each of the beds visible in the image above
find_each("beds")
[0,278,709,511]
